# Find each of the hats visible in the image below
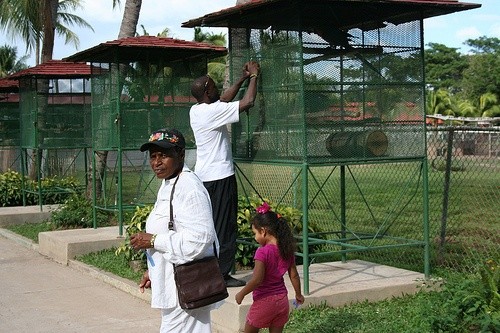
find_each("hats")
[140,127,186,152]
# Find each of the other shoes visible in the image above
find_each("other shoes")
[223,275,247,287]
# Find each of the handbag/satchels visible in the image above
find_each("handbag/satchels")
[175,256,229,310]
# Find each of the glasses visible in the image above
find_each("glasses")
[205,75,211,89]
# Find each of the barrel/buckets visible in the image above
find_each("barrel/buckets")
[326,129,388,157]
